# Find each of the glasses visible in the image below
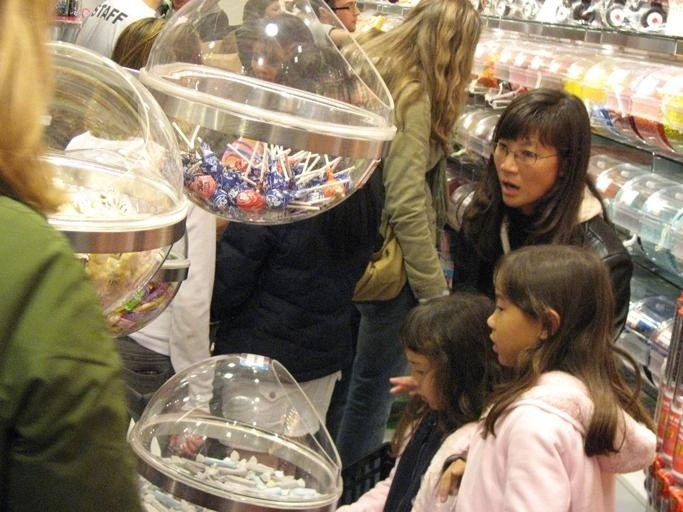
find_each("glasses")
[486,139,563,165]
[329,1,358,12]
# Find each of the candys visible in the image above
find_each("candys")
[140,450,317,512]
[476,55,683,153]
[76,252,175,335]
[172,119,354,214]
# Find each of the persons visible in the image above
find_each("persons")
[389,84,636,414]
[313,1,483,511]
[329,291,508,510]
[454,243,657,510]
[1,0,145,512]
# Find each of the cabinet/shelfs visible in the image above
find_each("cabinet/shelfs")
[442,11,683,506]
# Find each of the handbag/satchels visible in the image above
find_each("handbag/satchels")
[351,224,410,303]
